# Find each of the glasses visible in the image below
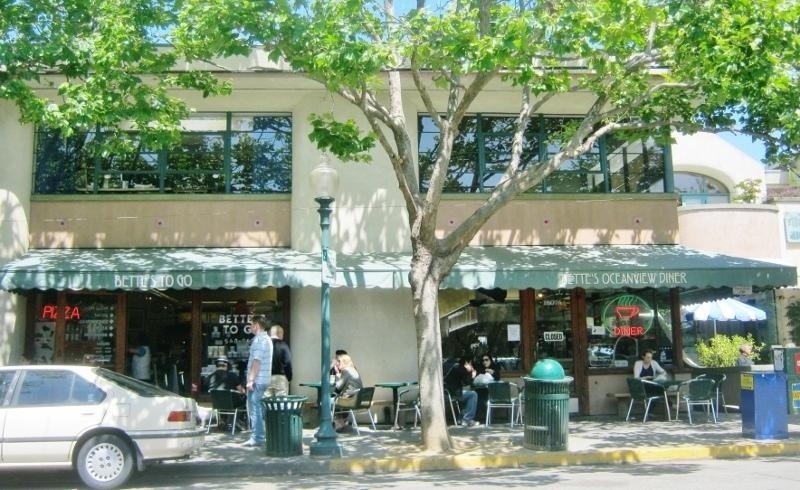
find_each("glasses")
[482,359,490,362]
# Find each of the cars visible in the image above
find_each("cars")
[1,363,208,490]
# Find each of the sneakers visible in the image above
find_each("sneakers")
[242,439,264,446]
[457,419,480,426]
[646,413,657,418]
[334,419,351,432]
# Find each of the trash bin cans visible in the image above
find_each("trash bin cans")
[523,359,575,452]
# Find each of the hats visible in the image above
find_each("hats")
[215,355,229,364]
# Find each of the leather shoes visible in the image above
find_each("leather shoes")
[226,424,233,431]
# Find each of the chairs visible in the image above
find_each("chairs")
[674,378,716,424]
[485,381,522,428]
[393,387,422,430]
[696,373,728,419]
[444,389,462,426]
[335,387,377,437]
[625,378,674,424]
[206,388,247,436]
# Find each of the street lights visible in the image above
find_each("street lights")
[309,150,341,456]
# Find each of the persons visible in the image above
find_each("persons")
[330,349,350,386]
[262,325,292,397]
[332,354,362,433]
[127,333,152,384]
[206,354,247,431]
[477,354,501,381]
[443,356,481,426]
[243,316,273,446]
[633,348,667,419]
[735,345,755,366]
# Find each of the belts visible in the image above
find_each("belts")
[272,370,285,374]
